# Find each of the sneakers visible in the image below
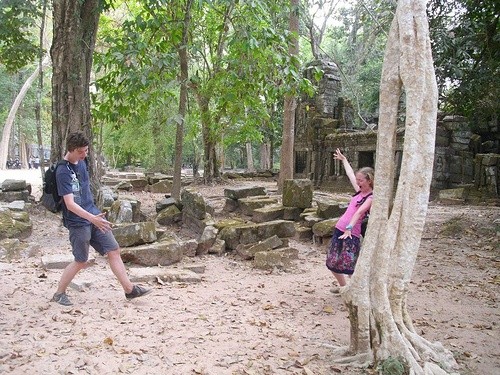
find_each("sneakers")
[48,292,75,312]
[330,283,348,294]
[126,283,155,302]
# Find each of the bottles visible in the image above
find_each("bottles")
[71,173,82,205]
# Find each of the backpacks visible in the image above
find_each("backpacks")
[43,160,78,213]
[360,214,369,237]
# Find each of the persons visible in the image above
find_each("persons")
[50,131,154,304]
[326,148,373,293]
[6,153,40,169]
